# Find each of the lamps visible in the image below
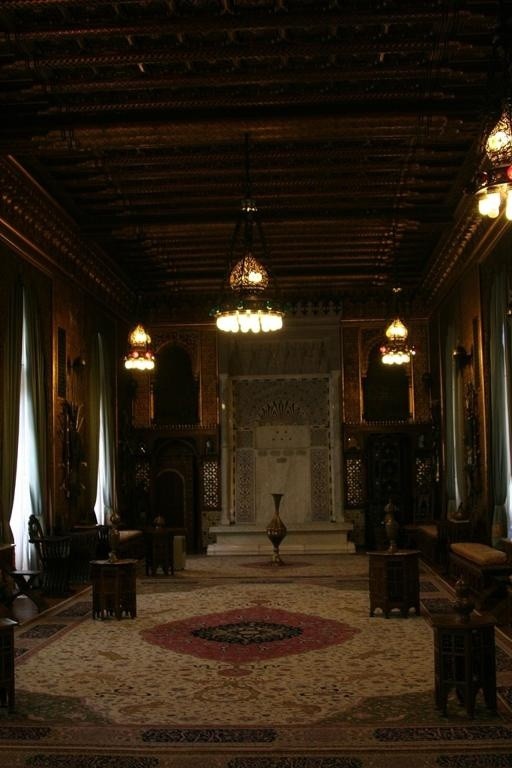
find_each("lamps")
[379,218,416,367]
[122,322,159,372]
[472,91,512,222]
[207,131,288,338]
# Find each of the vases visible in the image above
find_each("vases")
[266,493,286,563]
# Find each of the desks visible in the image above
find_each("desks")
[427,614,499,718]
[144,527,172,575]
[0,618,19,711]
[367,549,423,617]
[90,559,139,618]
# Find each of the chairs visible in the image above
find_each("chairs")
[0,514,111,613]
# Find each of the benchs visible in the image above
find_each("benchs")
[419,525,438,559]
[446,539,508,603]
[118,530,142,548]
